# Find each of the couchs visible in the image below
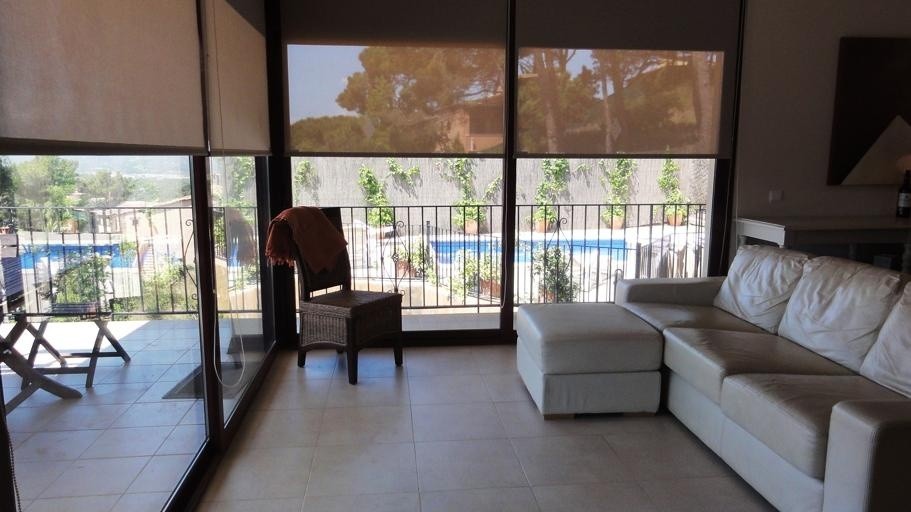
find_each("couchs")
[613,242,911,511]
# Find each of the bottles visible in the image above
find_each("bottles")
[896,170,911,217]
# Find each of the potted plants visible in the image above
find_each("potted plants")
[396,242,438,285]
[356,157,421,238]
[432,157,693,235]
[448,247,588,303]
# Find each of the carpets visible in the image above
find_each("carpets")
[161,360,260,402]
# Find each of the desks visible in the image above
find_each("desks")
[734,212,911,276]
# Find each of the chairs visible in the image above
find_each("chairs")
[293,205,406,386]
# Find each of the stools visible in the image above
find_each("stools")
[515,300,666,420]
